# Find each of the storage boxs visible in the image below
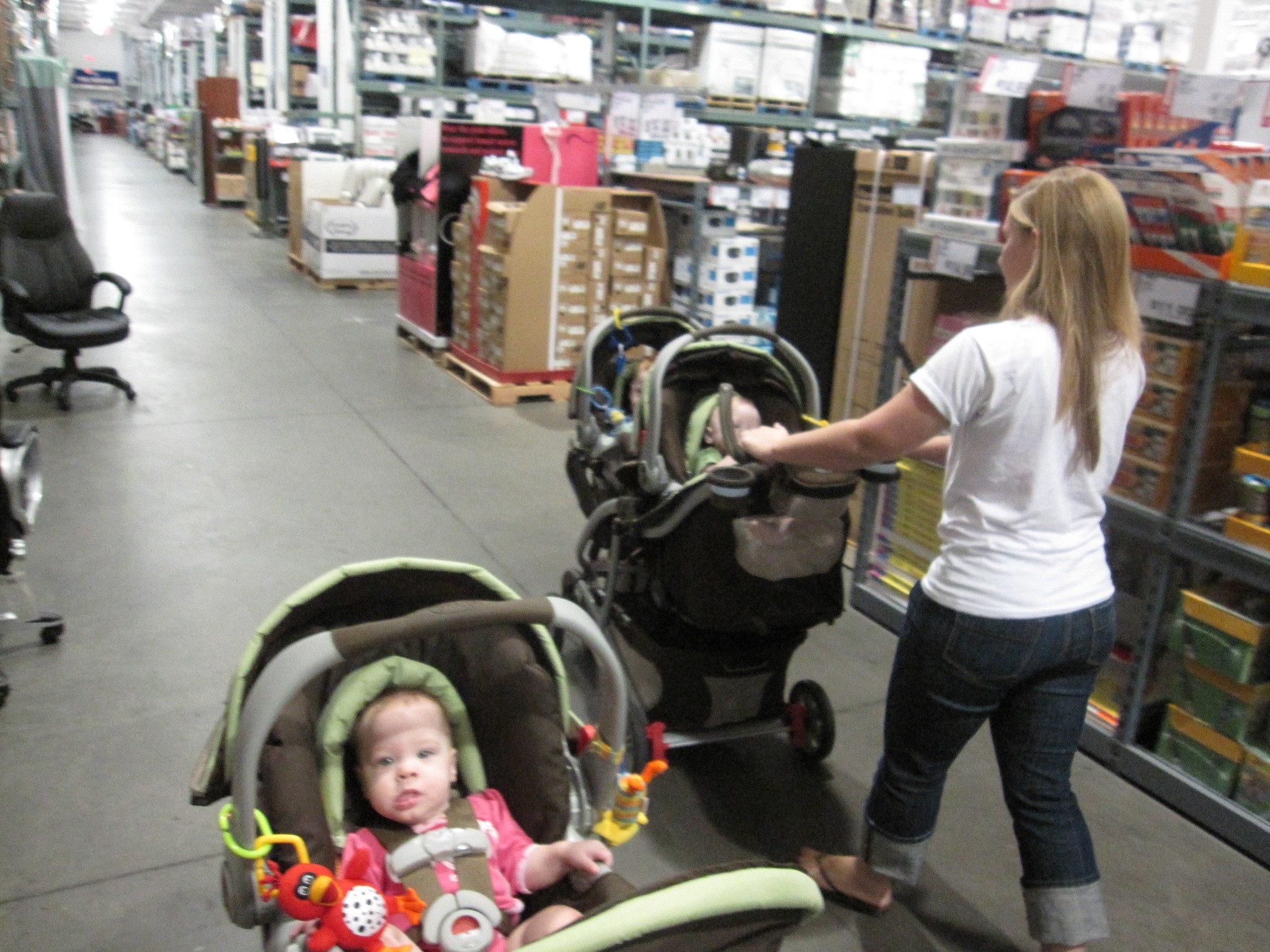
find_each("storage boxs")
[362,0,1268,817]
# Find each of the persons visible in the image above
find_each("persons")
[628,360,654,408]
[695,395,762,474]
[736,166,1147,952]
[343,689,614,952]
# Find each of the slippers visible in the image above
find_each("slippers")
[795,851,889,915]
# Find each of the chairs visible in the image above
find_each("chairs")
[0,191,136,411]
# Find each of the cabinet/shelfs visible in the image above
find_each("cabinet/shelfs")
[400,1,1172,409]
[850,225,1270,868]
[138,1,396,290]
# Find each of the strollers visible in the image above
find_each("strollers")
[182,551,830,952]
[544,308,902,780]
